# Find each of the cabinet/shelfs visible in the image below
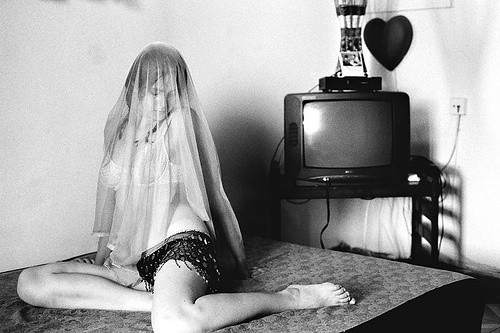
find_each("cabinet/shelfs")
[260,160,440,269]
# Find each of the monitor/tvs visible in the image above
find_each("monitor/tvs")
[284,91,411,188]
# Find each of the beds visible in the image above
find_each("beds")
[0,233,485,333]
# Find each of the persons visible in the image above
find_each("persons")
[15,43,356,332]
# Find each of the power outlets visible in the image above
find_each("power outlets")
[448,97,467,116]
